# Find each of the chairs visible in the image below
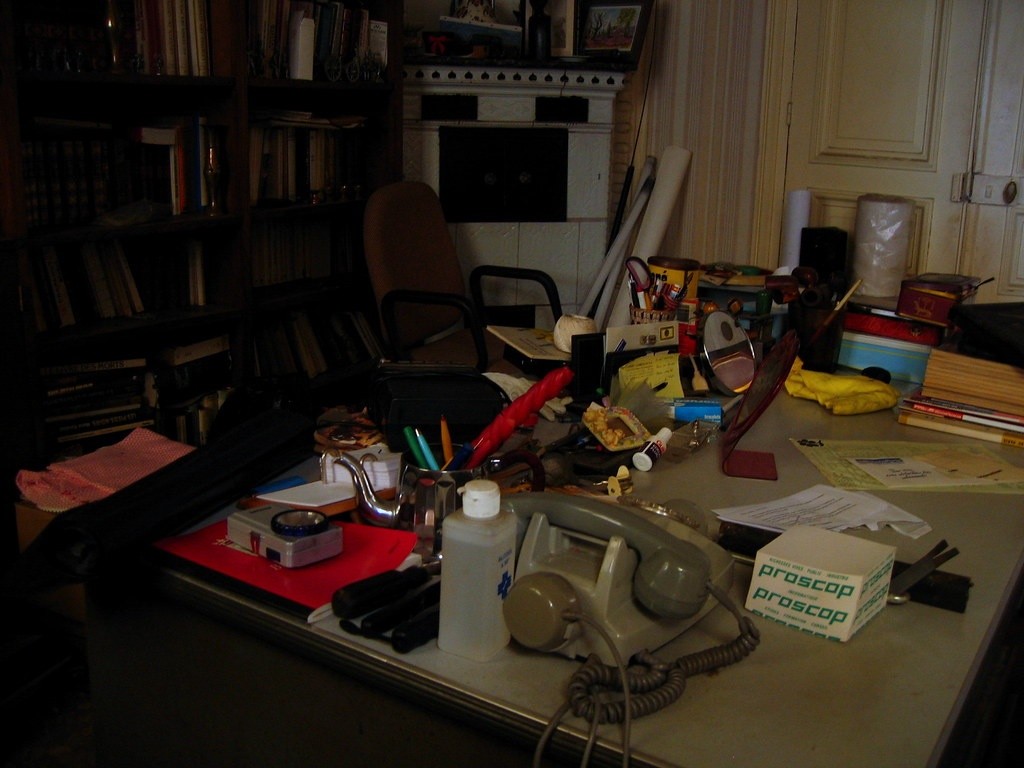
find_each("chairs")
[367,180,563,384]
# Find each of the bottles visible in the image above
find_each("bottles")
[438,479,518,657]
[632,427,673,472]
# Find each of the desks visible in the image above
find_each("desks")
[84,367,1024,768]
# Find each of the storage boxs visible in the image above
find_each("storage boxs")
[744,523,898,642]
[895,271,982,326]
[841,332,933,388]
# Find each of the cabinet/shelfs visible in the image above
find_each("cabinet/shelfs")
[0,0,405,590]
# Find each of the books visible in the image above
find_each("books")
[896,387,1024,449]
[147,512,423,622]
[0,0,386,458]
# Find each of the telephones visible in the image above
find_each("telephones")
[497,466,737,667]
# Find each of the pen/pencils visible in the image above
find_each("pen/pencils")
[405,414,476,470]
[630,273,684,310]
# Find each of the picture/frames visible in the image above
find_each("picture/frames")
[579,0,655,64]
